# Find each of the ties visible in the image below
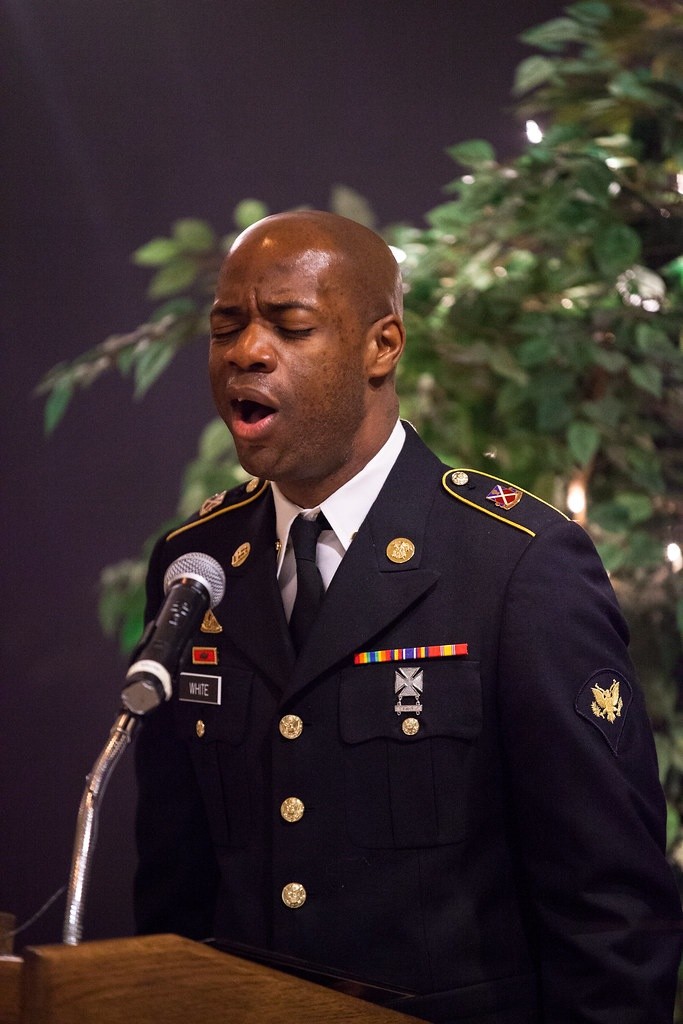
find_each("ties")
[282,510,333,634]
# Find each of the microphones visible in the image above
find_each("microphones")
[118,551,227,719]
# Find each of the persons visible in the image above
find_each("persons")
[128,204,680,1022]
